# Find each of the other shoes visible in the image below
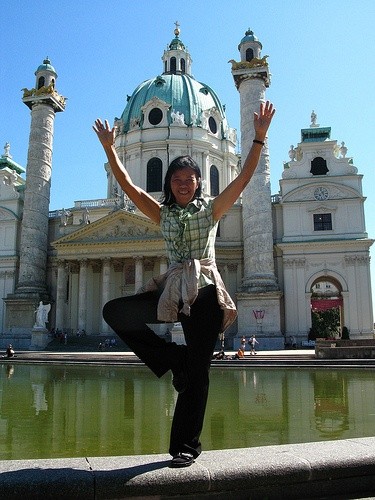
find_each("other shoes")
[171,451,193,468]
[172,342,189,393]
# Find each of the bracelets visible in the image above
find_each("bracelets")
[253,139,264,145]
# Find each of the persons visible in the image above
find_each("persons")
[50,327,69,345]
[248,335,260,355]
[286,335,298,348]
[92,101,276,468]
[34,301,48,327]
[240,335,246,355]
[96,336,115,351]
